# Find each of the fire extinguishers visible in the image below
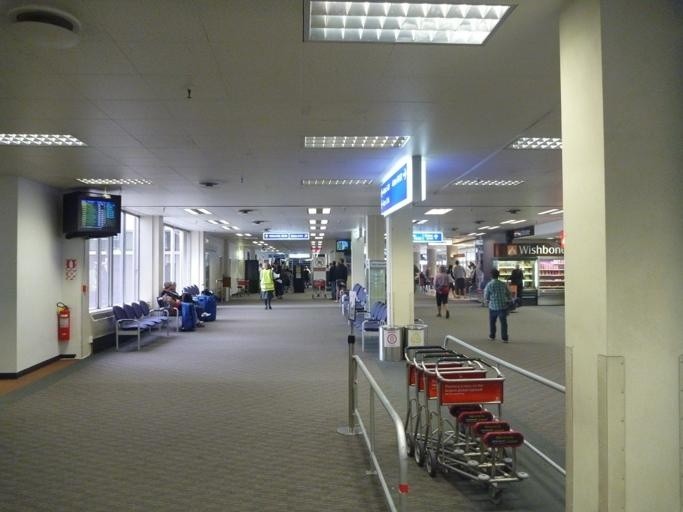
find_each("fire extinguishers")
[55,302,71,340]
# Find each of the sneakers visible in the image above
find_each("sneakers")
[264,306,272,310]
[446,310,450,319]
[437,314,442,318]
[193,310,212,328]
[488,336,495,340]
[502,338,509,343]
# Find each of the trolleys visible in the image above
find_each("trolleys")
[236,277,250,297]
[474,286,489,302]
[399,340,528,505]
[310,278,326,301]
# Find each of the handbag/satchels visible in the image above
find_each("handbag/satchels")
[507,297,517,311]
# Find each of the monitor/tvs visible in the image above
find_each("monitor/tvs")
[63,192,121,233]
[336,240,350,252]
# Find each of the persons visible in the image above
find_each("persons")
[507,264,522,300]
[170,281,211,319]
[424,266,433,290]
[466,262,477,299]
[419,271,427,293]
[483,269,512,343]
[434,265,450,320]
[447,264,459,299]
[258,256,348,310]
[452,260,467,299]
[156,280,206,327]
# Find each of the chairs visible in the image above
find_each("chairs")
[113,284,199,352]
[341,282,387,352]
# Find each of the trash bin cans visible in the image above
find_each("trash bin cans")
[378,325,426,361]
[220,287,231,303]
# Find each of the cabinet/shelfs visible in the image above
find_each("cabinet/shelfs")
[493,256,564,306]
